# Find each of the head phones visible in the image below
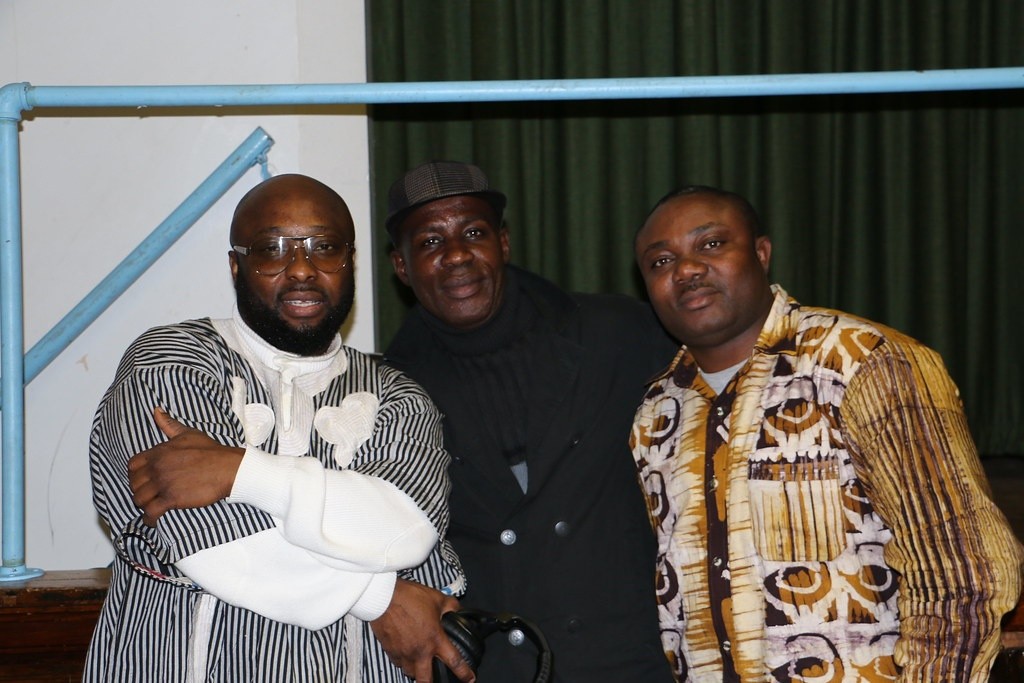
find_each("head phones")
[437,611,553,683]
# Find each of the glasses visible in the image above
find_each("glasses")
[231,234,354,277]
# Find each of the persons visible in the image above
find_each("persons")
[627,183,1023,683]
[386,161,679,683]
[82,173,478,683]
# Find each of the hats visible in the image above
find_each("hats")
[384,160,506,238]
